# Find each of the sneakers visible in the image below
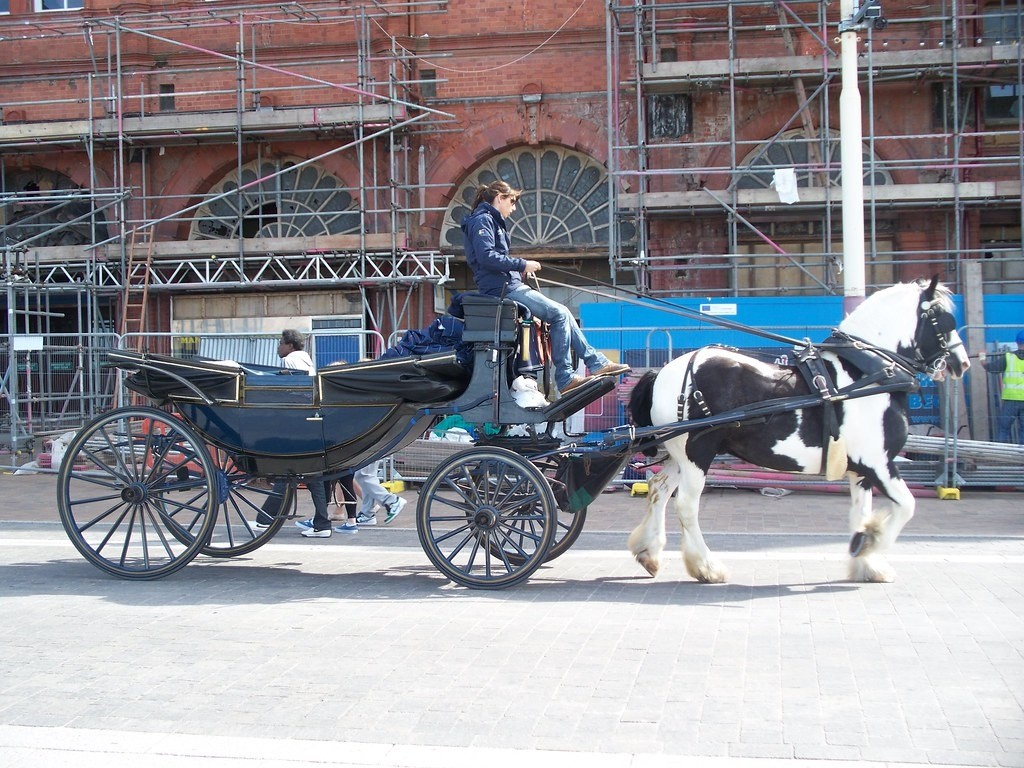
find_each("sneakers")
[384,495,407,524]
[300,524,331,537]
[560,376,595,396]
[589,360,631,378]
[247,519,270,533]
[355,510,376,525]
[295,518,315,531]
[334,523,357,534]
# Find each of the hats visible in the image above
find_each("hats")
[1015,329,1024,344]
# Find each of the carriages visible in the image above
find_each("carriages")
[56,272,974,591]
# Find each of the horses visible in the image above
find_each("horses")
[629,272,971,584]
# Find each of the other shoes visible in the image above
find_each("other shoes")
[328,513,344,521]
[373,503,381,513]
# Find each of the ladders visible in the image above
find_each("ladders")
[113,226,155,423]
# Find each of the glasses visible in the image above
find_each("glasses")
[507,194,517,205]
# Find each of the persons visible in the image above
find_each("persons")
[978,330,1024,445]
[246,328,406,538]
[460,179,630,395]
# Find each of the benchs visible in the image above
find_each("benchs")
[461,294,518,332]
